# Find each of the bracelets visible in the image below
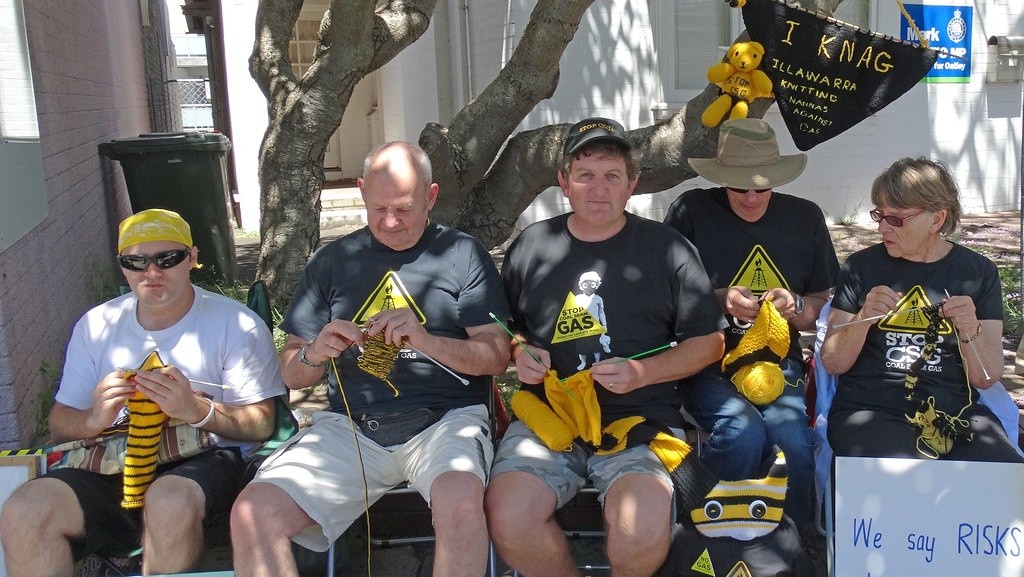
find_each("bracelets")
[188,398,214,428]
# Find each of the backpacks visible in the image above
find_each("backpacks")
[669,513,818,577]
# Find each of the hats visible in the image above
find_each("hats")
[563,116,631,156]
[687,118,808,191]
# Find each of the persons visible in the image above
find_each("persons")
[820,156,1023,463]
[663,119,840,534]
[229,139,510,577]
[0,209,287,577]
[484,118,730,577]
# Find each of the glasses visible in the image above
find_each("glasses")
[726,186,772,193]
[869,208,927,227]
[119,246,189,273]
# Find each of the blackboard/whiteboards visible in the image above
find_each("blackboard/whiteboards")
[835,456,1024,577]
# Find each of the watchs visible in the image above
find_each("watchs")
[299,340,329,368]
[790,292,804,321]
[958,322,983,344]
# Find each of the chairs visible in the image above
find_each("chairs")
[92,279,300,577]
[509,479,612,577]
[327,371,510,577]
[809,294,1024,577]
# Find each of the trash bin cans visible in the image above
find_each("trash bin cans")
[99,130,238,289]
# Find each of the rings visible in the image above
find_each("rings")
[610,383,613,388]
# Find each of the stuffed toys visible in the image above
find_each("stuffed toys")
[701,41,772,127]
